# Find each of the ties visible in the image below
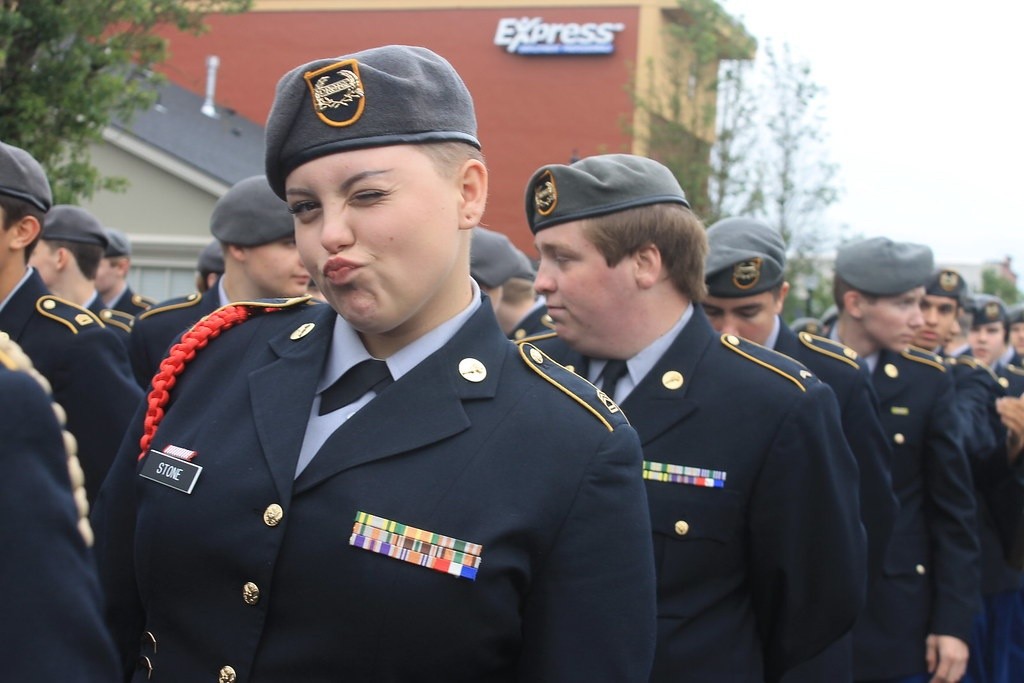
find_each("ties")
[602,358,629,401]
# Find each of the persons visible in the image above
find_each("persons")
[92,43,656,683]
[0,141,1024,681]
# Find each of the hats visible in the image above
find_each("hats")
[524,151,690,236]
[961,292,1008,326]
[703,215,785,298]
[926,270,967,307]
[40,204,110,247]
[102,226,131,257]
[0,141,53,212]
[467,224,539,291]
[198,241,224,271]
[835,235,933,294]
[1009,308,1024,323]
[264,45,480,202]
[208,174,293,245]
